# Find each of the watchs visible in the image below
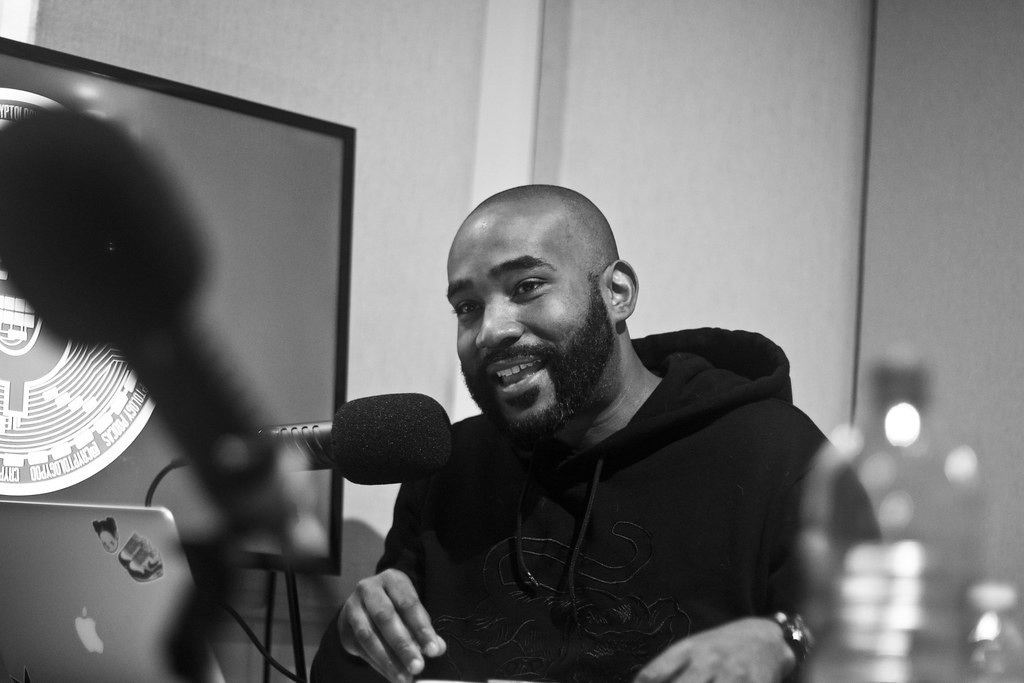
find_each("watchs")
[771,610,811,665]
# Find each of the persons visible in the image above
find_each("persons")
[310,184,881,683]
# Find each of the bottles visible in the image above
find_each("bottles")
[790,364,990,683]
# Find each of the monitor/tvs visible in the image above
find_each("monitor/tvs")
[0,498,230,683]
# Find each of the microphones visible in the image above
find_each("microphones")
[201,393,454,489]
